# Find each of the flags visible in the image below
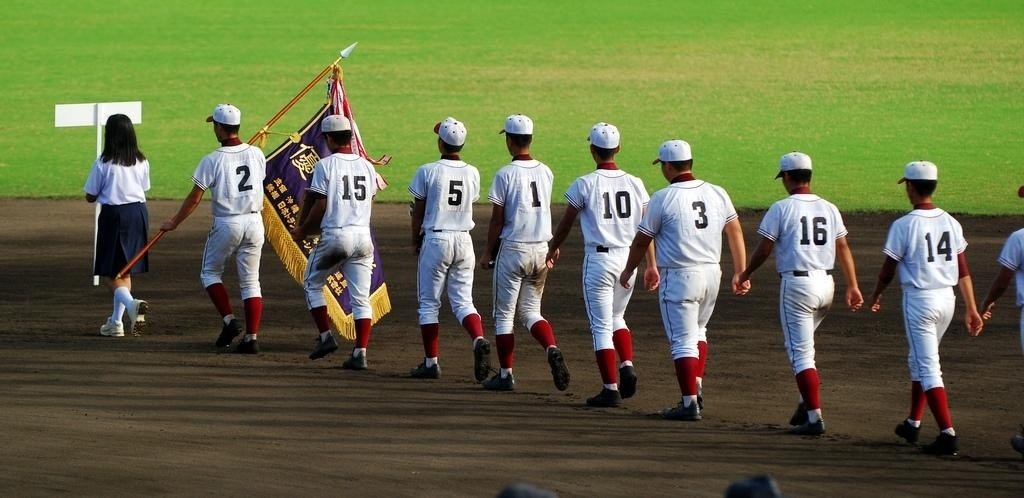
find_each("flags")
[263,59,390,342]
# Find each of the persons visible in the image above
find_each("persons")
[738,151,864,437]
[84,114,150,338]
[620,138,752,421]
[292,115,376,371]
[481,112,571,391]
[161,103,265,355]
[544,123,660,407]
[982,185,1023,456]
[872,161,984,457]
[406,116,491,381]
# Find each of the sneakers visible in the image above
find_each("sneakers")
[232,336,260,354]
[791,418,826,435]
[128,298,149,337]
[343,347,367,369]
[922,432,959,456]
[410,339,704,423]
[100,317,125,337]
[309,332,338,360]
[895,420,921,445]
[216,319,242,348]
[790,402,809,426]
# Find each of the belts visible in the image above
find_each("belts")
[794,268,836,279]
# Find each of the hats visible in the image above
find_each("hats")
[206,103,241,126]
[316,115,352,139]
[774,151,813,180]
[897,160,938,185]
[432,114,695,164]
